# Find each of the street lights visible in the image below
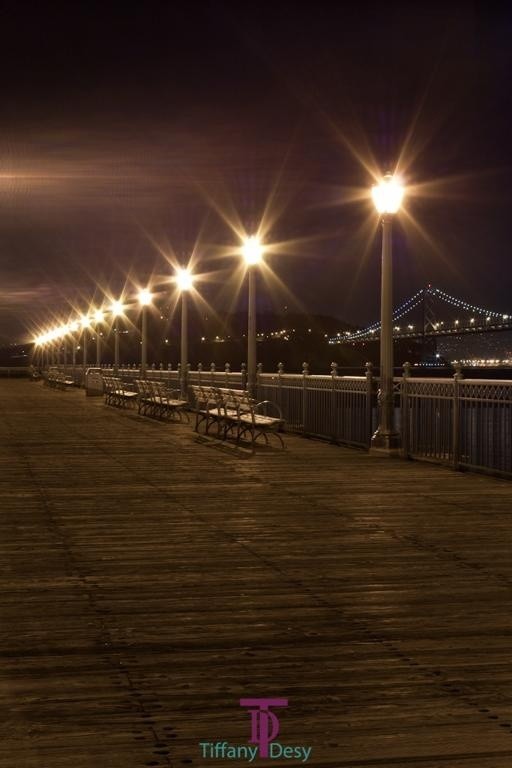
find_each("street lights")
[136,286,151,379]
[175,270,195,392]
[370,170,409,461]
[240,234,266,405]
[28,293,123,389]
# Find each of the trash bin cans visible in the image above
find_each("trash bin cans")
[85,368,104,397]
[370,399,413,449]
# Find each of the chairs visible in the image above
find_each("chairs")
[48,372,74,391]
[192,385,285,449]
[135,379,190,424]
[101,376,138,408]
